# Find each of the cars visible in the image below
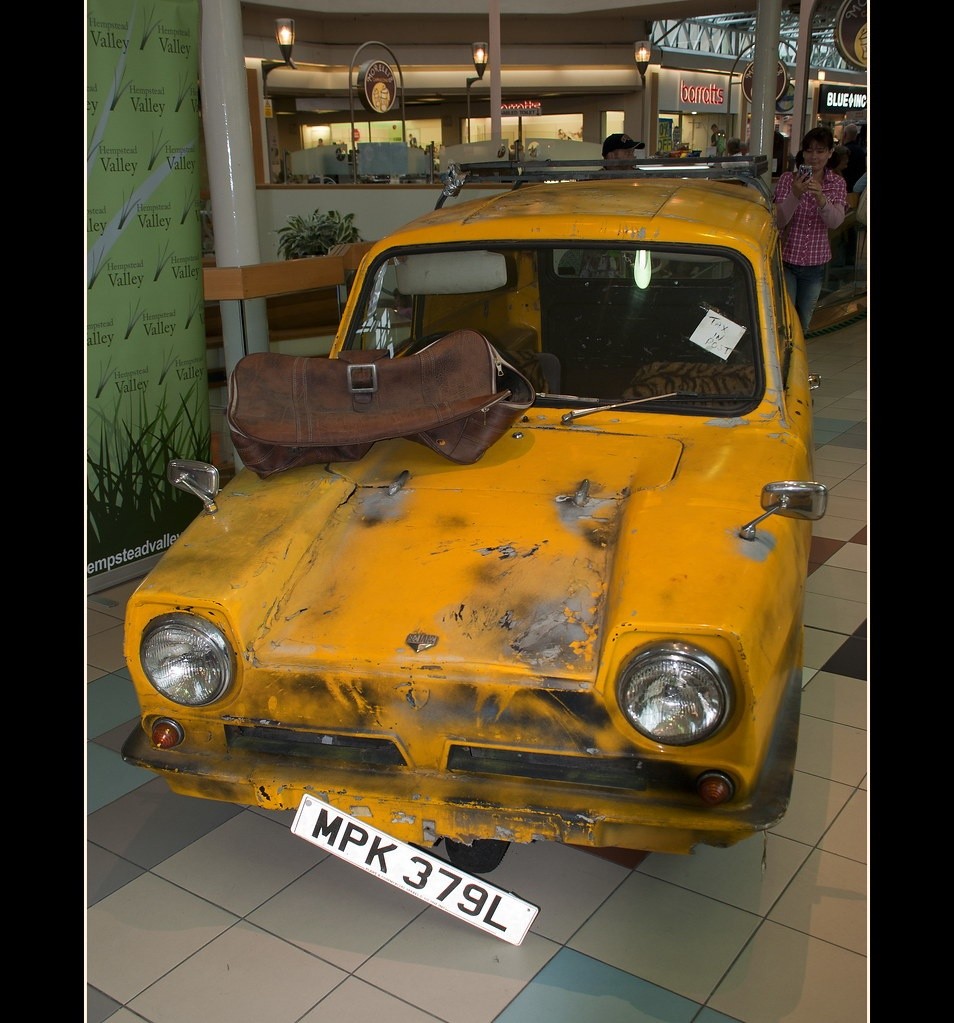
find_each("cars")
[116,156,828,947]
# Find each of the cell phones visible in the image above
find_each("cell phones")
[799,165,812,181]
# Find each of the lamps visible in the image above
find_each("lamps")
[263,16,297,96]
[635,41,651,87]
[466,42,490,143]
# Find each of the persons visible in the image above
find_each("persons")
[774,127,848,342]
[578,134,646,279]
[710,123,750,168]
[795,123,867,280]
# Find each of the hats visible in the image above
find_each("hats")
[602,134,645,156]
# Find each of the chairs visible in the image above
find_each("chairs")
[625,287,756,411]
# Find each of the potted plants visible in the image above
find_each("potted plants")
[275,206,366,260]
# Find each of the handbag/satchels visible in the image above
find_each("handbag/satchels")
[225,328,536,480]
